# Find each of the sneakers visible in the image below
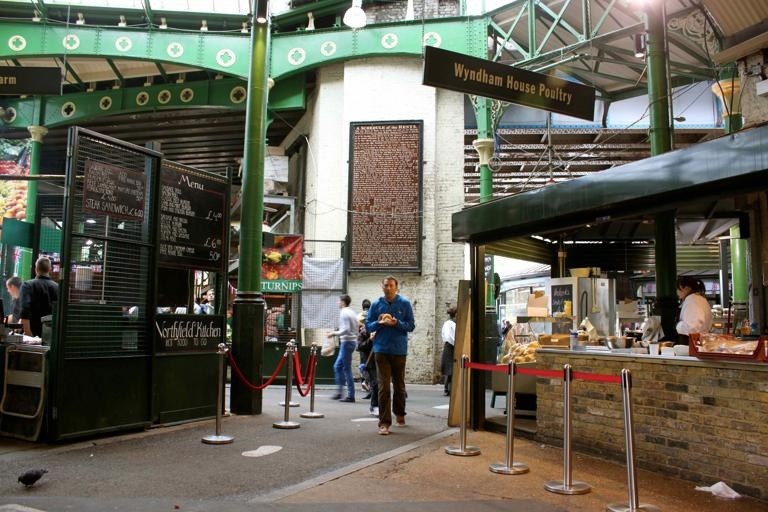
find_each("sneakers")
[378,425,390,435]
[332,382,380,416]
[395,414,405,426]
[443,390,449,396]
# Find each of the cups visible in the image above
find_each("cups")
[649,341,660,355]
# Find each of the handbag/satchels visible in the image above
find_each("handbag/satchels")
[359,363,371,381]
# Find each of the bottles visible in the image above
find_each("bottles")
[570,330,578,351]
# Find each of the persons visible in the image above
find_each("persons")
[276,304,286,331]
[500,320,512,335]
[157,307,187,315]
[194,282,216,315]
[227,310,233,325]
[663,277,713,344]
[326,294,356,403]
[4,277,24,324]
[357,323,380,416]
[440,306,457,395]
[123,306,139,317]
[365,276,415,435]
[357,300,372,391]
[19,256,59,338]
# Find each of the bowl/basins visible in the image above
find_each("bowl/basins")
[605,336,633,349]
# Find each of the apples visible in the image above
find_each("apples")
[0,184,27,224]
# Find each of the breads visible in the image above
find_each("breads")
[501,332,590,363]
